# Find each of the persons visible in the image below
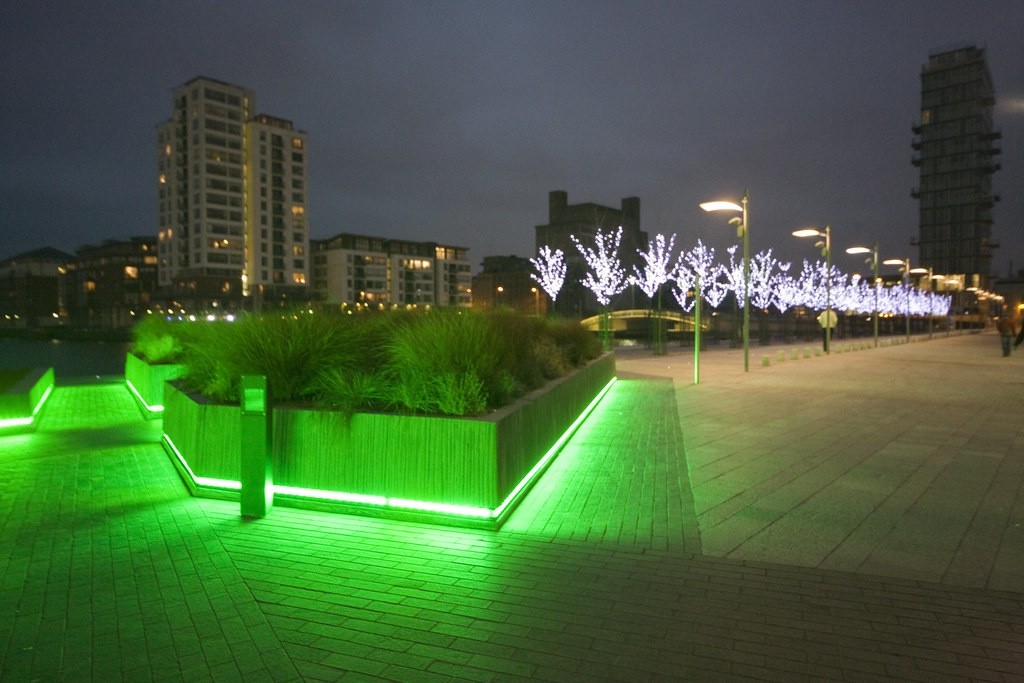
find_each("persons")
[819,306,838,352]
[1010,321,1024,352]
[996,310,1018,356]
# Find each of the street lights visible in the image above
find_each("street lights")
[845,246,879,348]
[909,266,1003,340]
[792,225,830,353]
[699,196,749,372]
[883,257,909,344]
